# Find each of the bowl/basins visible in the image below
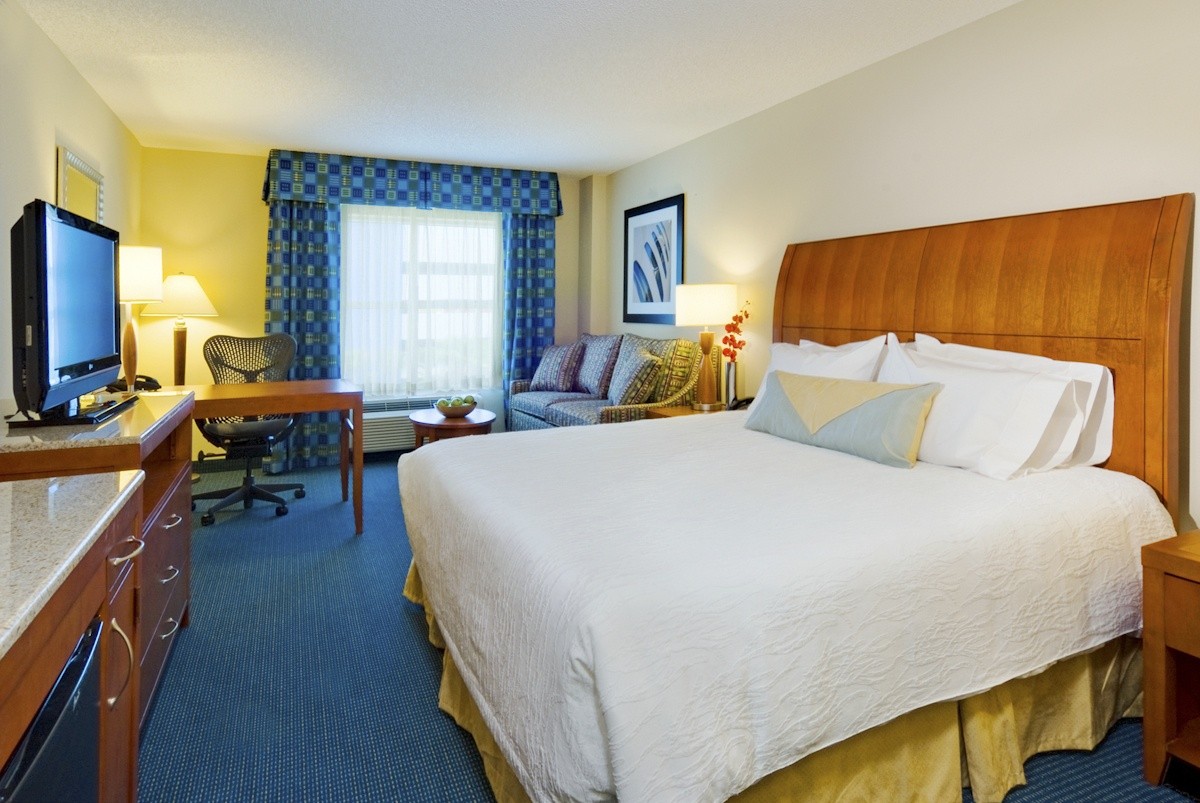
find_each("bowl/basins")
[434,401,477,418]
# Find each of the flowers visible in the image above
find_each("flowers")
[724,302,748,360]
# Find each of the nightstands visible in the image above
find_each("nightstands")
[1141,527,1200,784]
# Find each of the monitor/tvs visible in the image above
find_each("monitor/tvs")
[5,198,140,429]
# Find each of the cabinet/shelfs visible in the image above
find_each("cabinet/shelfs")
[0,392,191,803]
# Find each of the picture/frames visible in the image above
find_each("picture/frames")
[57,149,103,223]
[623,192,685,323]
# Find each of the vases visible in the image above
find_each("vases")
[725,362,738,408]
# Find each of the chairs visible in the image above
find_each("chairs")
[192,335,306,525]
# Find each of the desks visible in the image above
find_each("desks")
[410,409,495,448]
[161,379,364,531]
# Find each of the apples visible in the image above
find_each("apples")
[438,396,474,406]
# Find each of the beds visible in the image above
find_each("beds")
[397,194,1195,802]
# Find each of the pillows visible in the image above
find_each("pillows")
[747,342,1117,470]
[533,334,662,406]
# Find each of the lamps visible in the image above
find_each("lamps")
[677,284,735,409]
[118,245,220,391]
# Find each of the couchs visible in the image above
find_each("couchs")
[506,329,720,433]
